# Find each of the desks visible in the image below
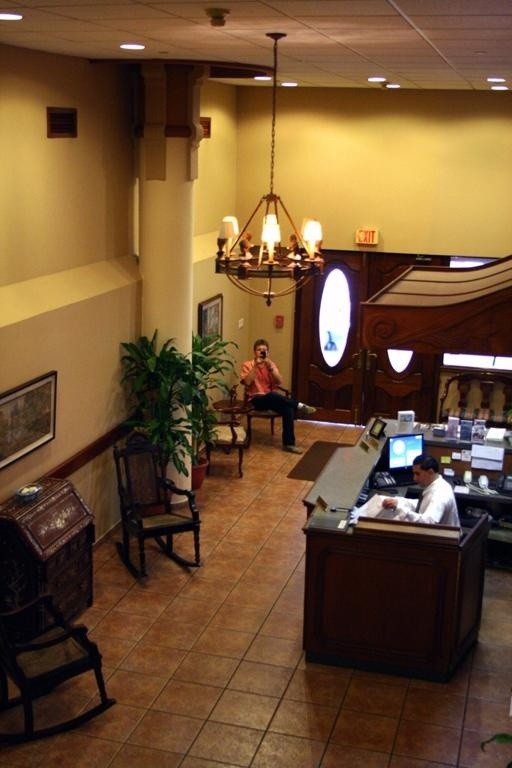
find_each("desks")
[212,398,255,455]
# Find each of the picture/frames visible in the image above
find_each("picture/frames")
[199,293,223,354]
[0,369,58,471]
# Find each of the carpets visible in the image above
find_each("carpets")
[287,438,356,482]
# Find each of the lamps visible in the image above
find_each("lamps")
[215,30,326,309]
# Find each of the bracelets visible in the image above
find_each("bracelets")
[269,367,274,373]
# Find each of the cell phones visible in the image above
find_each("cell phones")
[259,350,266,360]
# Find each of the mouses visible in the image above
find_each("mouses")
[453,478,461,485]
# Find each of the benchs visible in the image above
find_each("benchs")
[438,372,512,433]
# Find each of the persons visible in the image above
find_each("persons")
[382,453,463,542]
[240,337,317,455]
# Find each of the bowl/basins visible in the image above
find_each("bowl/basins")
[16,483,41,502]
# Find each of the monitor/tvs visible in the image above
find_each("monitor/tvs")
[388,433,426,485]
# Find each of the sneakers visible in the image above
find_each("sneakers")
[299,404,316,414]
[283,446,303,454]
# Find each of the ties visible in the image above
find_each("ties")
[415,493,423,513]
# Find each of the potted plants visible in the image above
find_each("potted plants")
[118,327,210,516]
[191,329,240,490]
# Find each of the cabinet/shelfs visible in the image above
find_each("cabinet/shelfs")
[0,477,94,625]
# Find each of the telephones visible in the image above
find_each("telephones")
[478,475,489,488]
[372,471,398,491]
[463,470,473,484]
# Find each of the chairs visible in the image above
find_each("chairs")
[229,377,290,437]
[198,401,246,479]
[0,592,117,749]
[112,430,204,581]
